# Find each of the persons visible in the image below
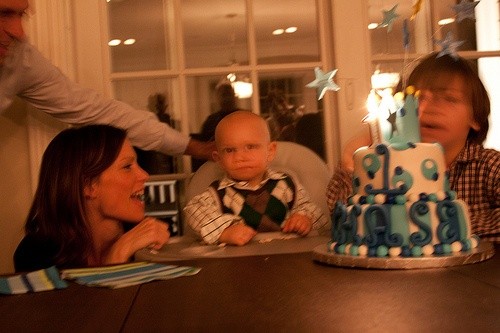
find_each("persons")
[325,51,500,245]
[133,78,325,175]
[182,110,330,248]
[0,0,222,161]
[12,124,171,274]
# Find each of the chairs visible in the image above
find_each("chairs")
[181,141,334,238]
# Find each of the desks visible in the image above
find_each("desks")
[1,239,500,333]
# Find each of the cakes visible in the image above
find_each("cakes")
[326,86,482,258]
